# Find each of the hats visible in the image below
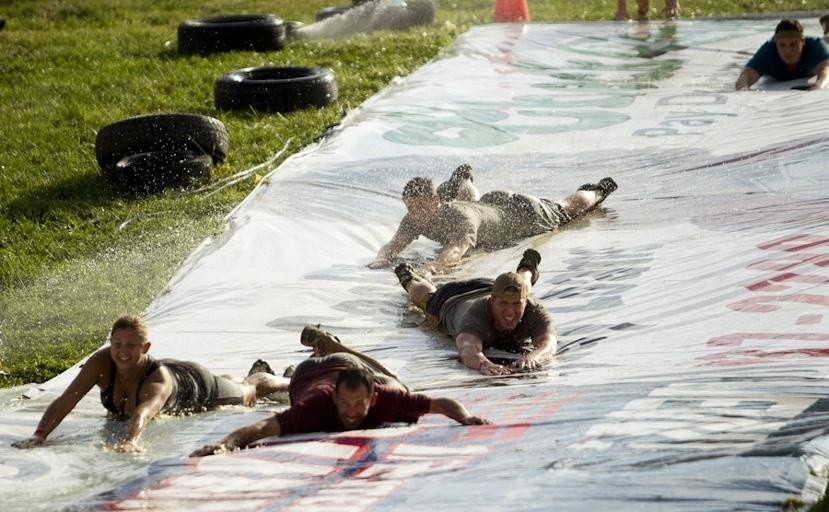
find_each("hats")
[493,272,529,300]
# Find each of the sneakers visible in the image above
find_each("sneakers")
[394,262,434,293]
[577,178,619,212]
[249,359,275,377]
[517,250,541,286]
[436,163,473,199]
[300,323,340,346]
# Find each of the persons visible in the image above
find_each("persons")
[188,321,490,459]
[614,0,627,20]
[394,249,557,375]
[493,0,530,22]
[367,162,618,273]
[735,13,829,91]
[636,1,680,20]
[11,315,294,454]
